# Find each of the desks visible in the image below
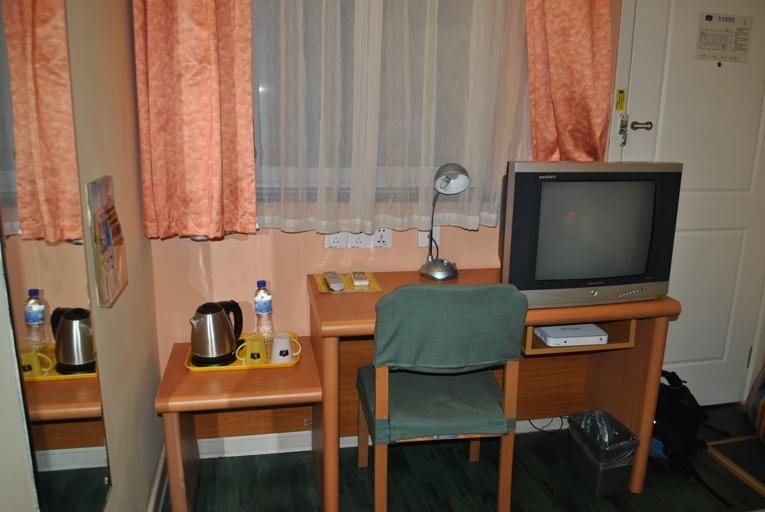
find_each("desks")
[153,267,683,512]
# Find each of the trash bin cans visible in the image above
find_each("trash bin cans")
[568,408,639,497]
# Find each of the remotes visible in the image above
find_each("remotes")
[351,271,369,286]
[324,271,345,293]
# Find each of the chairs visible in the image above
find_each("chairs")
[358,285,528,512]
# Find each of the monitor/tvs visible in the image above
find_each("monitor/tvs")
[497,162,684,309]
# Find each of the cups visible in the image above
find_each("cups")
[17,346,54,377]
[271,333,301,363]
[235,334,267,364]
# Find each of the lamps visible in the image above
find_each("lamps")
[418,163,472,281]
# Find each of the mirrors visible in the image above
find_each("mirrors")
[0,0,113,512]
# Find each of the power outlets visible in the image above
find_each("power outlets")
[372,228,392,248]
[348,232,370,248]
[326,232,346,248]
[417,226,440,247]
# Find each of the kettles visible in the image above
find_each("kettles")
[50,306,97,376]
[189,299,244,367]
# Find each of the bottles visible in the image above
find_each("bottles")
[255,281,274,343]
[25,289,47,363]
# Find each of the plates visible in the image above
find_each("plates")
[16,341,99,382]
[183,332,300,371]
